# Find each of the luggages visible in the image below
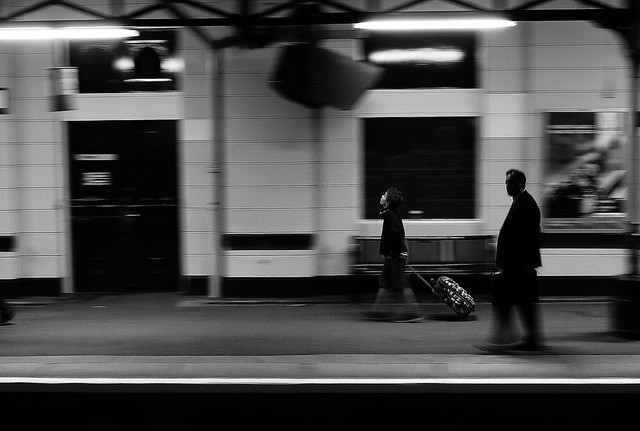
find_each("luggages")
[403,260,476,319]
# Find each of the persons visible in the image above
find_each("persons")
[358,188,426,324]
[474,169,549,356]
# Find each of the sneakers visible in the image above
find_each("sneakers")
[473,337,524,348]
[360,311,392,319]
[503,334,551,354]
[391,313,425,323]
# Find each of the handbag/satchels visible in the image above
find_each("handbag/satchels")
[490,269,539,306]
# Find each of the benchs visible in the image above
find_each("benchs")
[348,236,503,303]
